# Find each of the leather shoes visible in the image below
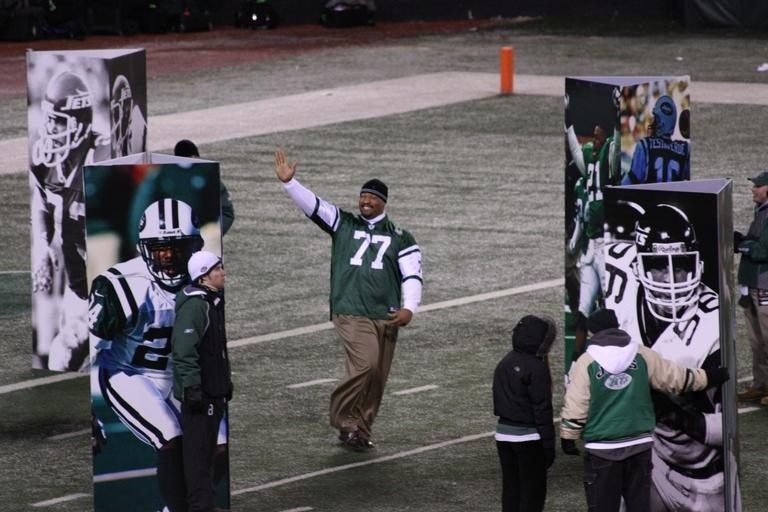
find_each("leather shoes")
[359,437,374,448]
[737,388,762,401]
[339,432,362,452]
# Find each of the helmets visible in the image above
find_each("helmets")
[633,203,706,323]
[110,74,133,150]
[603,199,647,245]
[187,251,222,282]
[646,95,677,138]
[33,71,93,168]
[136,198,204,286]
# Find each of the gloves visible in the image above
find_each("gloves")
[733,231,744,254]
[561,438,579,455]
[180,385,208,415]
[535,448,554,473]
[699,355,730,393]
[665,407,705,444]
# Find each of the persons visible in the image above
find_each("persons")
[733,170,768,408]
[26,71,112,374]
[574,205,740,510]
[270,148,425,450]
[174,140,235,234]
[92,197,226,512]
[564,90,621,366]
[561,306,729,511]
[108,75,145,159]
[490,312,555,512]
[177,251,231,510]
[620,94,689,186]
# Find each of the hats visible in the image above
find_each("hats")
[747,172,768,186]
[588,308,618,335]
[175,140,199,156]
[361,178,388,203]
